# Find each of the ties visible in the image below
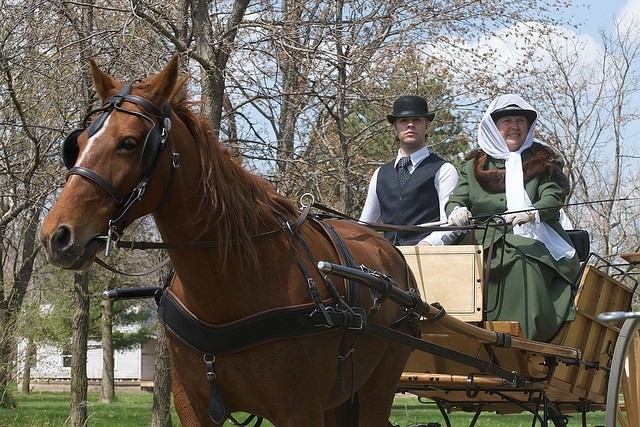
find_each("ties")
[397,155,412,188]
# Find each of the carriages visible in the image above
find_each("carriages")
[37,51,640,427]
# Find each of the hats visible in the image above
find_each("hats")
[387,96,435,125]
[491,104,536,124]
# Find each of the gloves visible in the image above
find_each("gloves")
[501,209,531,228]
[448,205,475,227]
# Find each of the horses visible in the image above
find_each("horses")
[39,53,420,427]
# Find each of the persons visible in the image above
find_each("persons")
[358,95,467,246]
[445,93,579,342]
